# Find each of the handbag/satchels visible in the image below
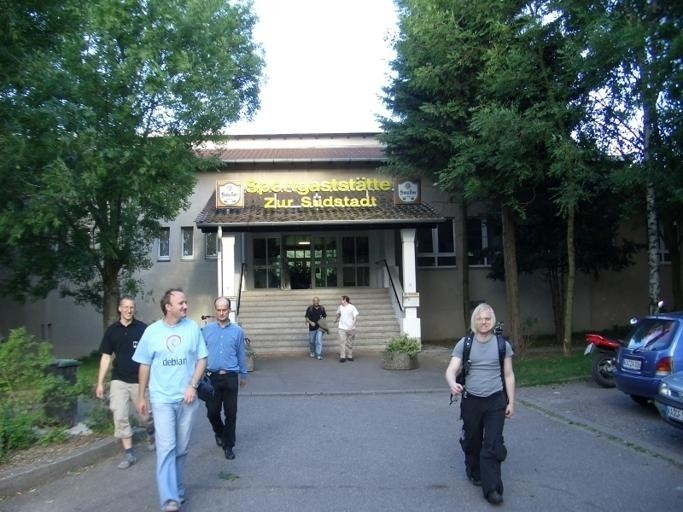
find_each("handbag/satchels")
[199,376,214,400]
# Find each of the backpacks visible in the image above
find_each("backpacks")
[457,330,505,391]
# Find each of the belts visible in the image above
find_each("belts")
[216,370,230,374]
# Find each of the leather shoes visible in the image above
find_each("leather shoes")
[216,433,235,459]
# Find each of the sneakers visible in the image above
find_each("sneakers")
[340,358,345,362]
[146,428,156,452]
[484,489,503,504]
[164,500,179,512]
[119,453,136,468]
[468,473,482,485]
[348,358,352,361]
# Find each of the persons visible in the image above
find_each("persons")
[445,303,516,504]
[333,295,360,362]
[95,295,155,469]
[305,297,327,360]
[131,286,209,512]
[202,296,247,459]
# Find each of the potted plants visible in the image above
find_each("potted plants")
[384,333,420,370]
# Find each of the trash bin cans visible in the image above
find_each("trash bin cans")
[43,358,83,430]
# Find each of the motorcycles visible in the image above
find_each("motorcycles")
[583,301,664,388]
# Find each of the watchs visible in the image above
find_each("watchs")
[189,382,198,389]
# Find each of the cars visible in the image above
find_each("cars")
[653,369,683,430]
[609,311,682,404]
